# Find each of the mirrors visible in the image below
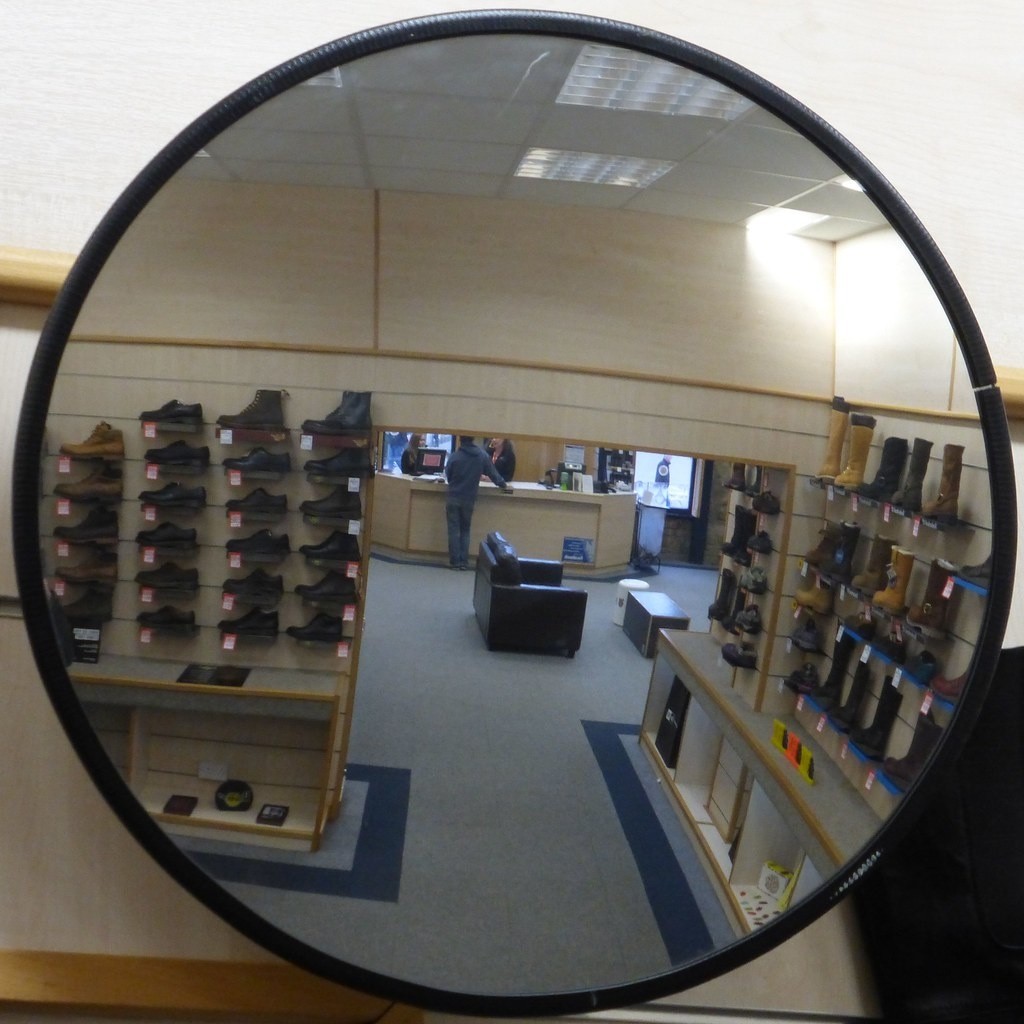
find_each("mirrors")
[0,0,1024,1024]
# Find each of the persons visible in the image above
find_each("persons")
[478,437,515,482]
[383,430,440,477]
[444,435,515,566]
[656,453,673,507]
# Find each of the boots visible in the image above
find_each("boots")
[707,396,994,784]
[217,389,289,429]
[301,391,373,436]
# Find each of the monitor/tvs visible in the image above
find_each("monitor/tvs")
[416,447,446,475]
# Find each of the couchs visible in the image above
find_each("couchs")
[471,531,588,661]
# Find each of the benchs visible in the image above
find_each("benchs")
[622,591,690,658]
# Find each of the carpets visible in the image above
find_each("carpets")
[165,764,412,903]
[579,719,738,969]
[654,548,673,566]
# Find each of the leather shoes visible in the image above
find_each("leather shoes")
[146,441,209,468]
[134,521,197,548]
[225,488,288,514]
[137,563,200,590]
[222,447,291,474]
[223,569,284,600]
[299,531,361,561]
[140,483,207,508]
[137,606,195,633]
[286,612,343,641]
[225,529,291,554]
[303,447,372,479]
[139,400,203,425]
[294,570,357,604]
[299,487,361,520]
[217,606,279,636]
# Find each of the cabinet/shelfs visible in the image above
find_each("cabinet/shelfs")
[637,628,882,941]
[42,375,373,856]
[799,474,986,799]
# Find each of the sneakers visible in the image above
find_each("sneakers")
[62,590,113,623]
[53,507,119,546]
[55,552,118,585]
[57,420,125,462]
[53,465,124,506]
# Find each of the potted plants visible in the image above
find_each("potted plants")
[707,461,787,674]
[632,544,655,569]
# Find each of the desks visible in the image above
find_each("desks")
[637,501,670,554]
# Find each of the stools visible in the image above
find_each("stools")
[613,578,650,628]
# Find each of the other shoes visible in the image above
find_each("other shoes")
[453,561,468,570]
